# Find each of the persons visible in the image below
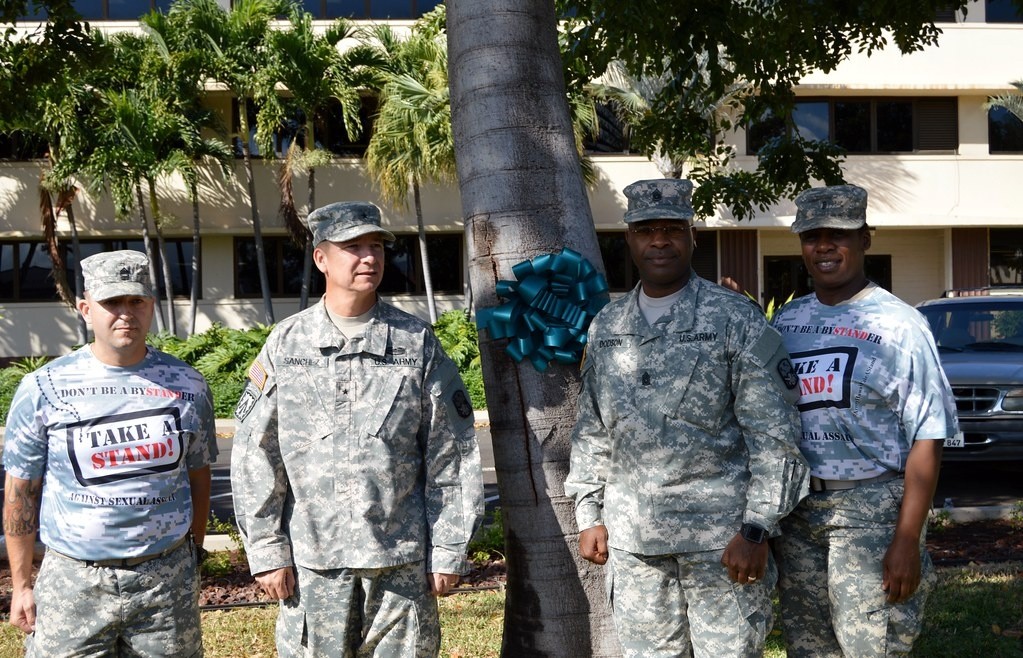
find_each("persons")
[3,250,219,658]
[771,186,960,658]
[231,202,486,658]
[563,179,811,658]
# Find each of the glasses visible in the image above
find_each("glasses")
[628,224,693,238]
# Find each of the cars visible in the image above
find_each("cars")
[914,296,1023,461]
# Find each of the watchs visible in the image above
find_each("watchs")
[740,523,769,545]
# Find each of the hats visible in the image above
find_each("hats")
[791,185,868,234]
[80,249,152,301]
[307,202,396,249]
[623,179,695,223]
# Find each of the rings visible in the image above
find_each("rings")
[748,576,756,580]
[450,582,456,585]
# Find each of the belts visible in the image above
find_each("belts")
[813,469,894,491]
[47,535,186,566]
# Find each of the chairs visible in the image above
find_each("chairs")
[942,315,974,348]
